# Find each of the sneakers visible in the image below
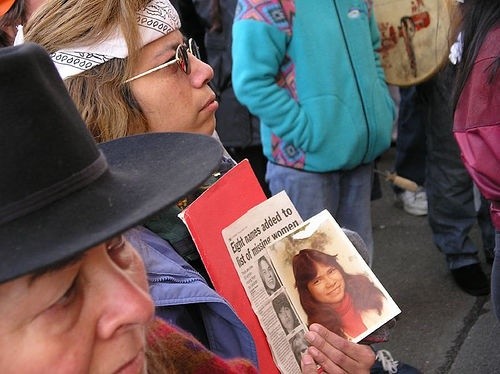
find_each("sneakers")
[397,184,429,216]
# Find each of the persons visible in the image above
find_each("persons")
[293,249,387,342]
[291,331,321,369]
[272,292,300,335]
[0,0,500,374]
[258,255,281,296]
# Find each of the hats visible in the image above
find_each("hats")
[0,40,225,281]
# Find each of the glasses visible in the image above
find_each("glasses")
[122,37,201,84]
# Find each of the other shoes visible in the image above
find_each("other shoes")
[448,262,491,297]
